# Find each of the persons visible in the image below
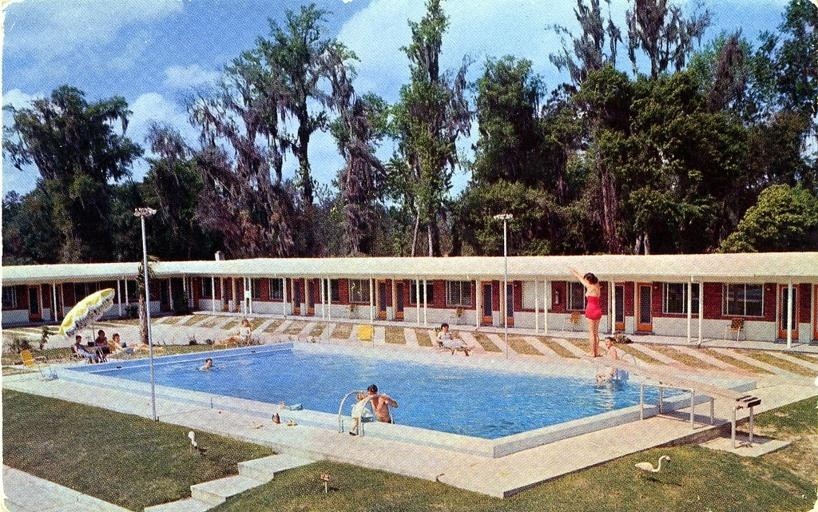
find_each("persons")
[202,358,214,370]
[596,337,617,384]
[593,385,615,414]
[112,333,131,355]
[205,337,243,348]
[71,335,96,363]
[436,322,475,352]
[95,330,110,358]
[566,263,602,357]
[356,384,398,423]
[241,319,251,346]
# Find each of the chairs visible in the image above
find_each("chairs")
[563,312,583,333]
[346,304,359,320]
[725,317,746,340]
[19,349,54,380]
[211,328,254,347]
[448,306,466,325]
[435,328,473,356]
[70,341,139,366]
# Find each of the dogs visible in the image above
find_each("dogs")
[595,367,617,389]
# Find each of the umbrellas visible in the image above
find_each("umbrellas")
[60,288,116,346]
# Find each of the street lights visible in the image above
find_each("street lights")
[135,206,157,421]
[494,215,514,362]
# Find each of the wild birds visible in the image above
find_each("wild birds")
[635,455,671,478]
[188,430,197,454]
